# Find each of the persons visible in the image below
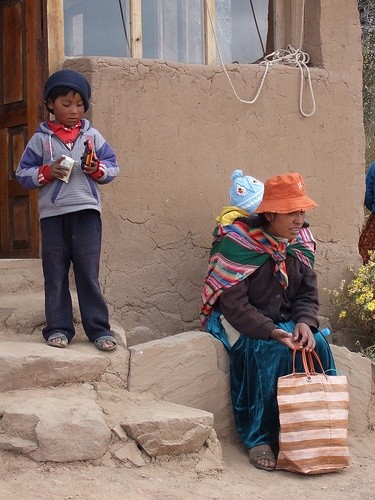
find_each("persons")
[209,171,337,471]
[217,169,265,225]
[15,70,120,351]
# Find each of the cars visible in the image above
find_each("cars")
[80,140,95,172]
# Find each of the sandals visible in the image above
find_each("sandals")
[94,335,117,351]
[48,332,68,348]
[249,443,276,471]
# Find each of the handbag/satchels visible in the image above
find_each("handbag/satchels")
[274,344,351,475]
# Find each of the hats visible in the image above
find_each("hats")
[43,69,92,115]
[228,169,267,216]
[252,172,319,216]
